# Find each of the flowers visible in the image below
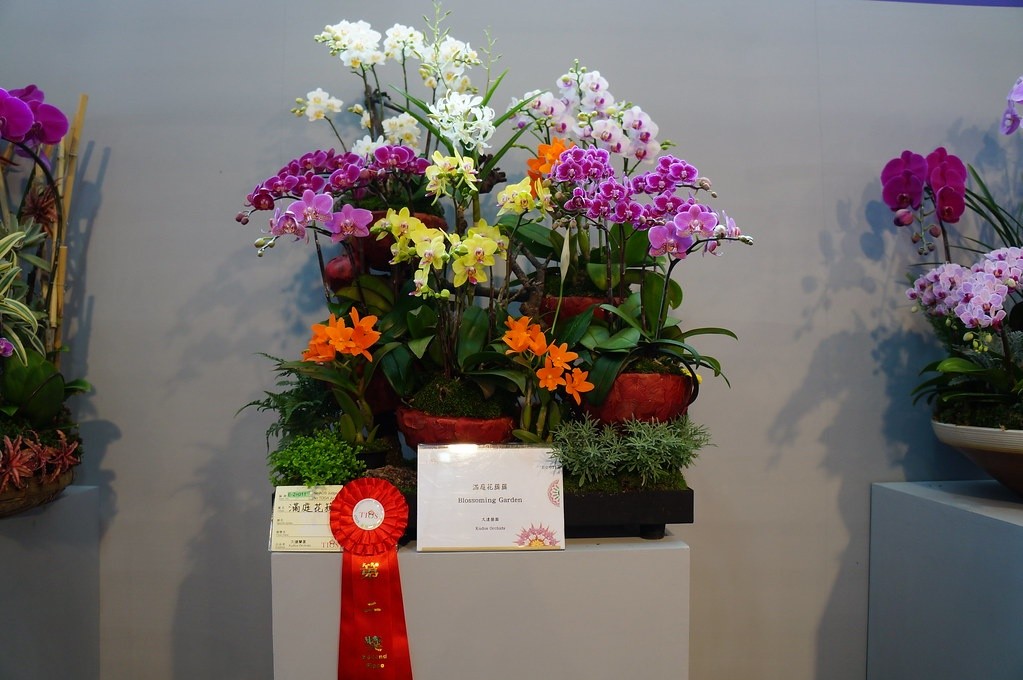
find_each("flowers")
[880,76,1023,430]
[0,83,98,443]
[231,0,752,434]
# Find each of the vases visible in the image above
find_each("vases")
[544,290,631,332]
[343,211,446,273]
[597,373,691,427]
[395,409,511,446]
[931,418,1023,493]
